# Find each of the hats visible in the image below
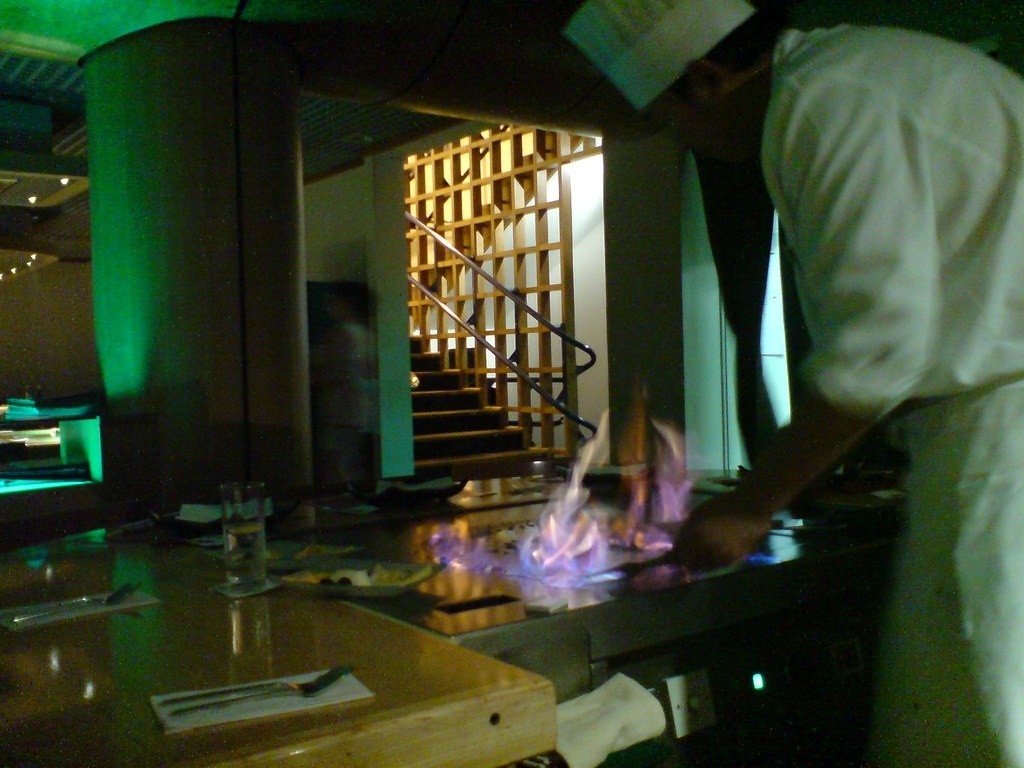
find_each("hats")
[565,0,759,113]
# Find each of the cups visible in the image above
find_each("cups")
[219,481,267,591]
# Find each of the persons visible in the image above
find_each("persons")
[568,0,1024,768]
[308,279,371,482]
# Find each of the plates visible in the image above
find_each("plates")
[272,556,447,598]
